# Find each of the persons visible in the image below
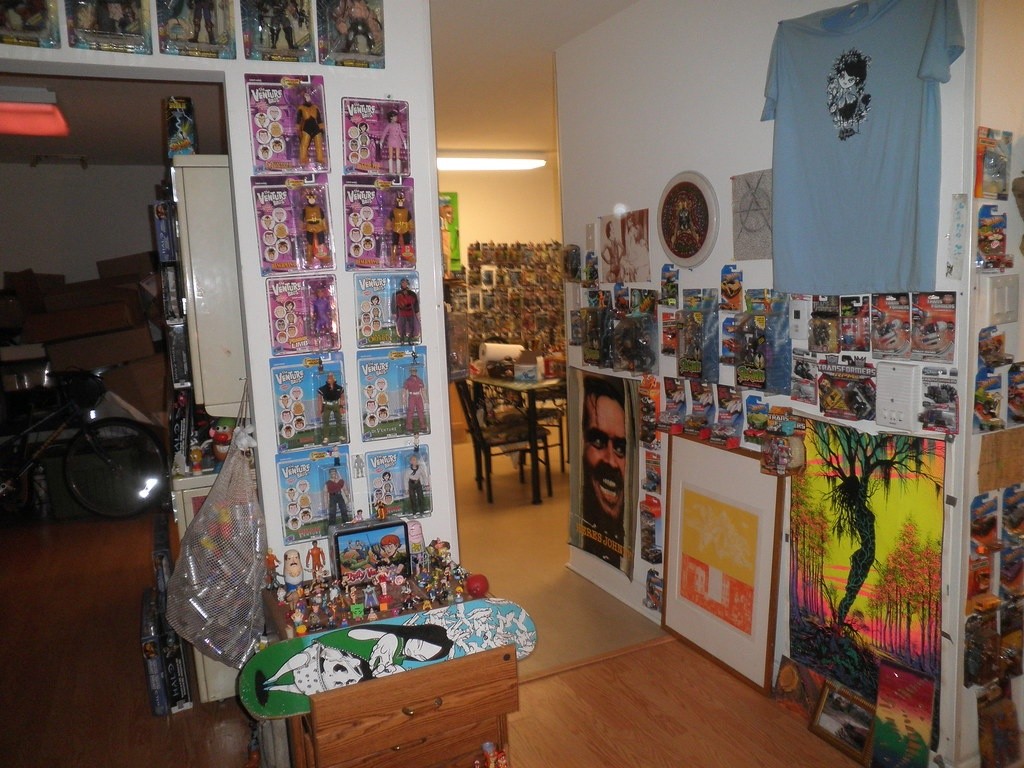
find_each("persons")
[580,374,628,568]
[0,0,383,55]
[601,212,649,283]
[296,92,429,527]
[265,538,326,592]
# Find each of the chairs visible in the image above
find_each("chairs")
[453,376,555,505]
[479,356,566,486]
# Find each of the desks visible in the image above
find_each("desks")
[468,371,570,506]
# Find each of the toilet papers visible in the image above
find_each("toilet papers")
[478,342,525,362]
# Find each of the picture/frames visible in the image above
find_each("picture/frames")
[807,677,881,768]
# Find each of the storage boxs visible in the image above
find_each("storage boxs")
[152,199,178,262]
[162,93,199,157]
[163,323,191,387]
[167,389,189,472]
[137,545,196,718]
[0,245,172,437]
[159,266,184,321]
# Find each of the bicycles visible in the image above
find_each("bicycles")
[0,365,170,529]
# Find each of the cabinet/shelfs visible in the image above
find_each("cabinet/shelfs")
[165,458,264,705]
[262,575,523,768]
[157,151,258,417]
